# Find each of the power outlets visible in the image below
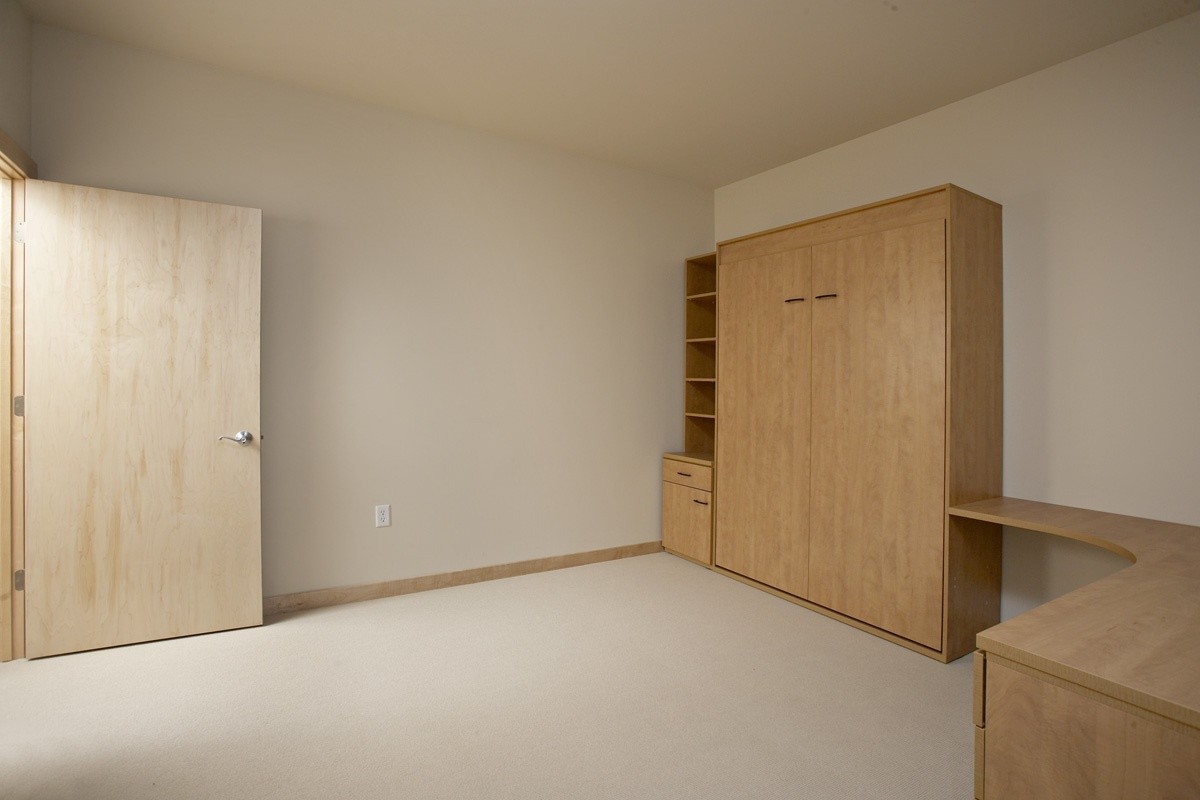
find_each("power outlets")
[375,505,390,527]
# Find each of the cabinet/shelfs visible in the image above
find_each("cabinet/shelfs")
[664,186,1200,800]
